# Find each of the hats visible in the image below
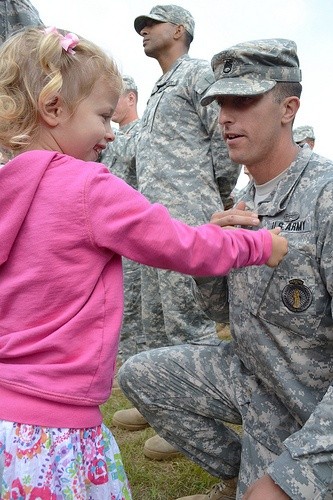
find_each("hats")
[293,124,315,144]
[200,38,303,106]
[121,74,138,91]
[133,4,195,40]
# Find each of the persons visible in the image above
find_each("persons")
[115,40,333,500]
[107,5,243,462]
[0,27,288,500]
[0,0,44,44]
[290,126,316,150]
[96,74,146,388]
[239,164,254,201]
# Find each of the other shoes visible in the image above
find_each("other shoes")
[173,479,236,500]
[143,433,185,460]
[112,407,151,430]
